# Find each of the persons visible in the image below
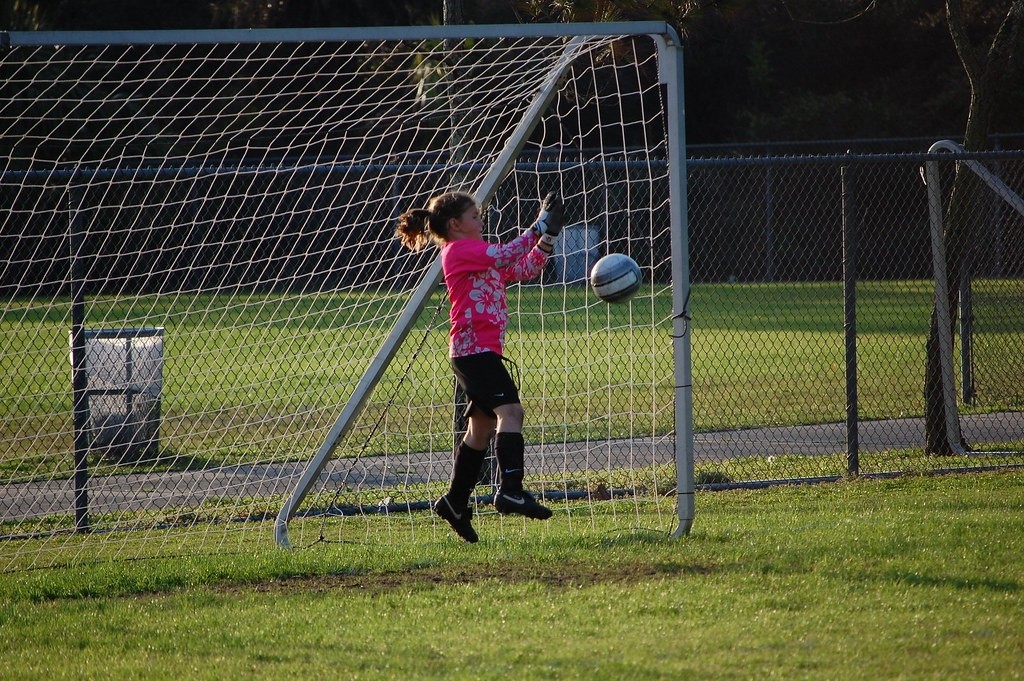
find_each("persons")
[395,189,572,543]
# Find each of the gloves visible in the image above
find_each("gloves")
[529,192,572,247]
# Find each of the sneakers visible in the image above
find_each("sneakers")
[432,494,479,543]
[494,489,552,520]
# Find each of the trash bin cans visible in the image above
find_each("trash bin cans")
[68,326,166,460]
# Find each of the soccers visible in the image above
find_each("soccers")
[591,254,643,304]
